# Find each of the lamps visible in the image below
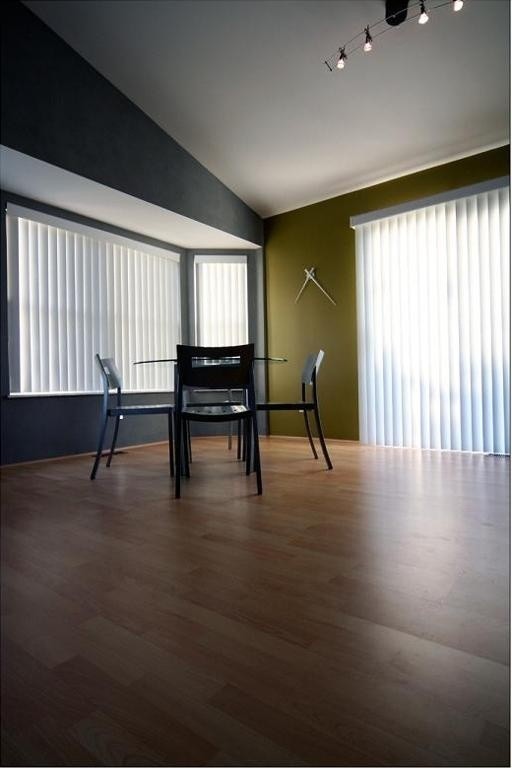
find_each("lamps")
[324,0,468,75]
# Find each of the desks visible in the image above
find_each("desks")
[131,355,290,480]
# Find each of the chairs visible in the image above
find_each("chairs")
[171,338,265,502]
[82,352,184,481]
[181,388,244,463]
[244,345,335,478]
